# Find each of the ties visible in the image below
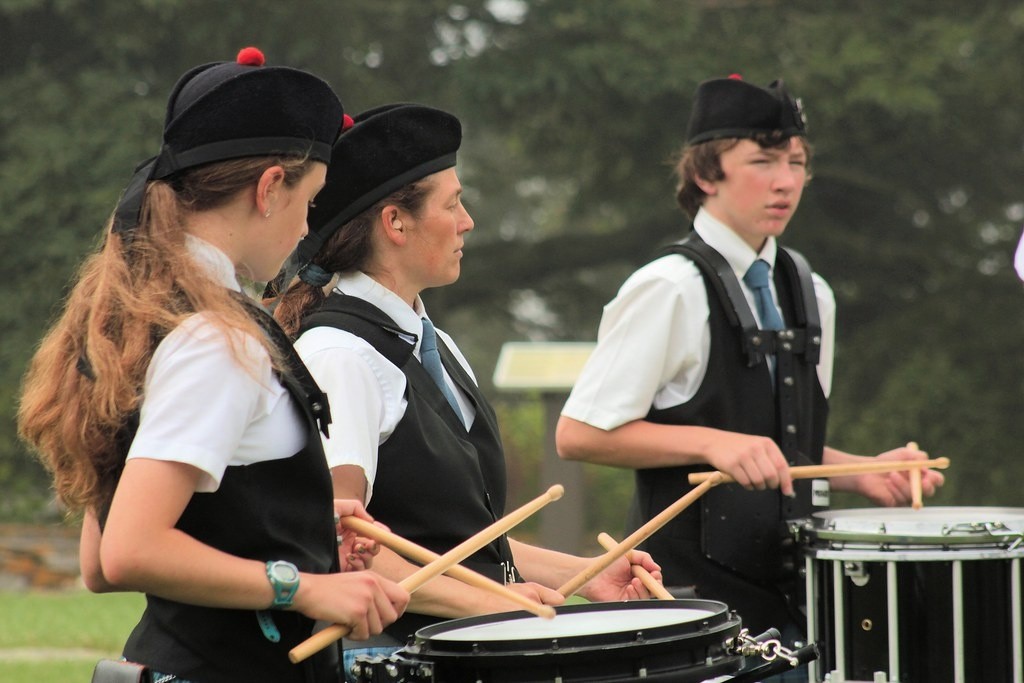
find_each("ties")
[743,259,787,403]
[420,318,467,432]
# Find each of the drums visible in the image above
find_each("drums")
[349,652,434,683]
[798,503,1024,683]
[406,597,749,683]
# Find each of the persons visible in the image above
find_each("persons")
[553,74,944,682]
[20,48,409,683]
[289,103,664,653]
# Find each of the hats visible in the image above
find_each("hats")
[262,100,465,300]
[111,45,345,235]
[684,72,808,148]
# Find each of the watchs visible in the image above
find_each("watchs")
[258,560,299,642]
